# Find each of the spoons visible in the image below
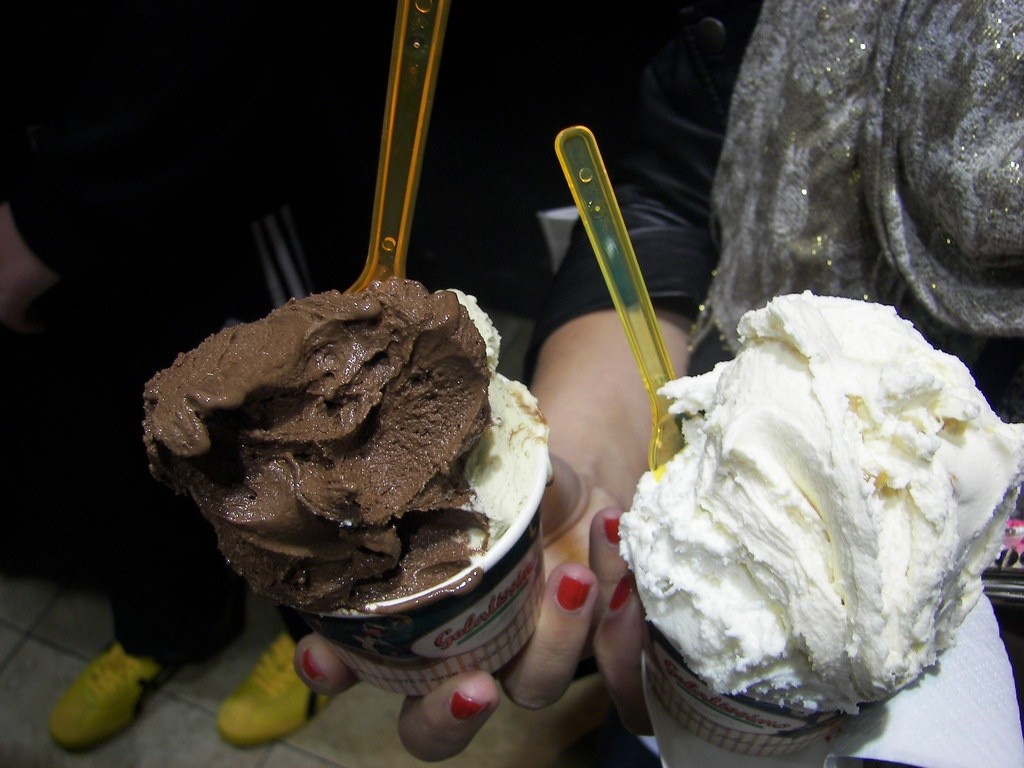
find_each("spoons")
[341,0,453,299]
[557,126,702,481]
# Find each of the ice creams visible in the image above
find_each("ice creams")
[140,277,550,693]
[615,288,1024,756]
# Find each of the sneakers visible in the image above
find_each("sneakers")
[215,631,335,751]
[48,638,176,751]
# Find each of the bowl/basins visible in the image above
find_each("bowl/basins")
[640,610,874,757]
[289,449,547,697]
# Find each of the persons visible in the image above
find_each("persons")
[0,0,346,752]
[295,0,1024,761]
